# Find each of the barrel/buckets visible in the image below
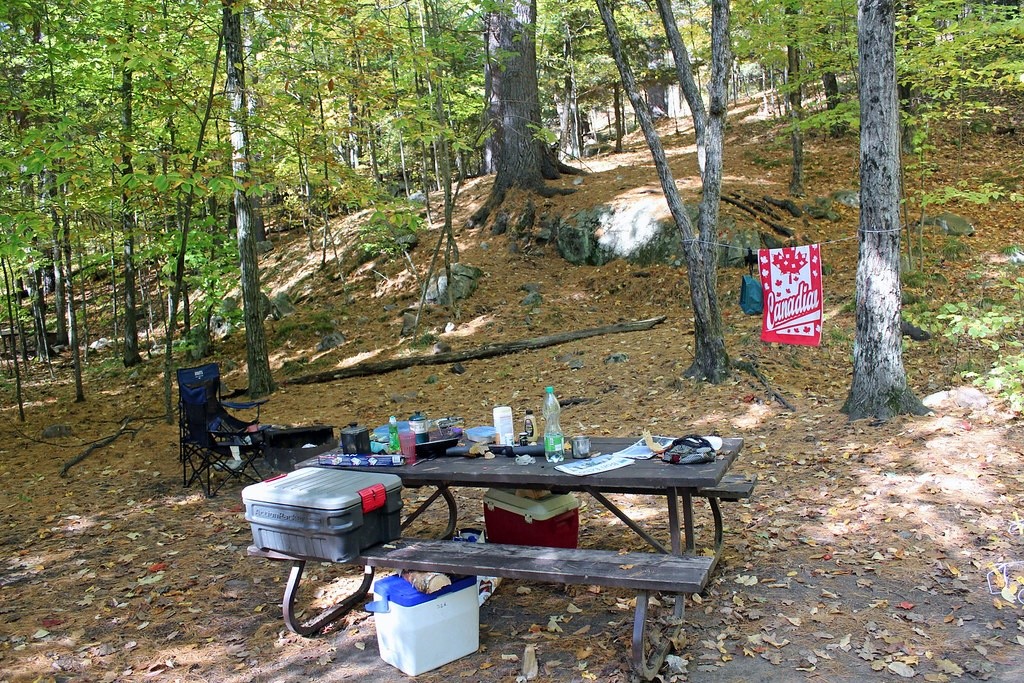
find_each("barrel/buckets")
[374,420,428,444]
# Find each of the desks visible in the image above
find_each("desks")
[294,435,744,623]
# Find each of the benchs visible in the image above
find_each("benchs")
[244,536,716,593]
[402,473,760,503]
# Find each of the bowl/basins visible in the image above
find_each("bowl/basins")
[465,425,496,444]
[416,437,460,457]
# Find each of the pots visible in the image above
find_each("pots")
[340,422,371,454]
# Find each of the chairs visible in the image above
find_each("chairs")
[178,362,272,497]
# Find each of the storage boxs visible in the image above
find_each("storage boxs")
[482,488,580,549]
[364,574,481,678]
[242,465,405,561]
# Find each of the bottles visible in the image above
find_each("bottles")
[388,416,400,452]
[519,410,538,446]
[408,411,430,444]
[542,387,565,463]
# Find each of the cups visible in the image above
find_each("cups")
[399,431,416,464]
[493,406,514,447]
[571,436,591,459]
